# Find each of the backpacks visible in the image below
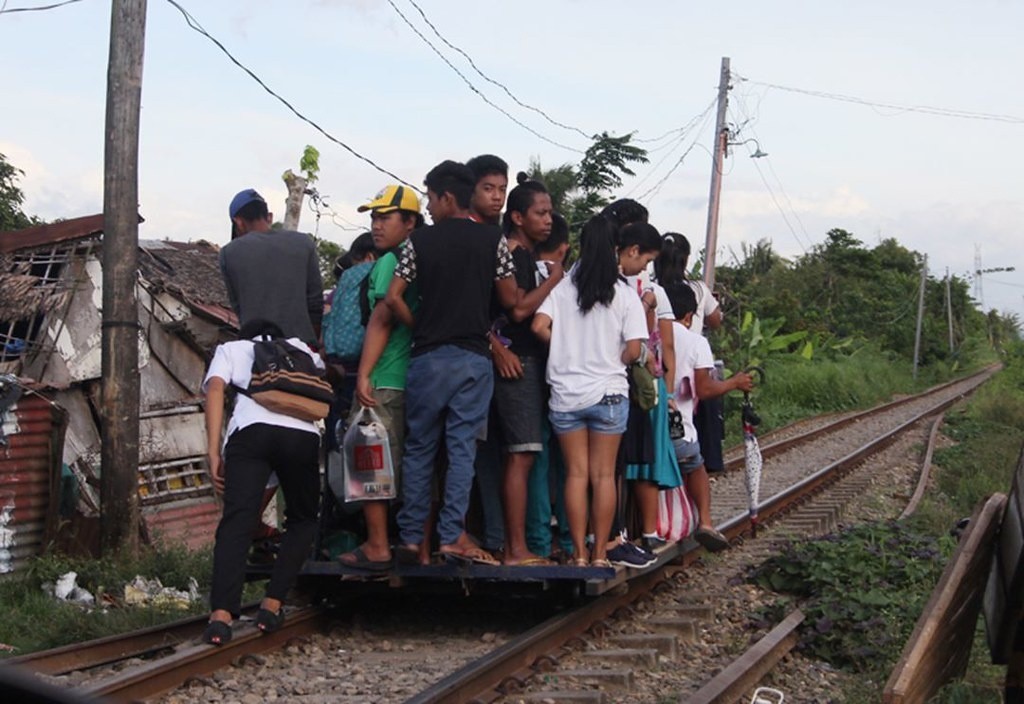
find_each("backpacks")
[232,336,335,421]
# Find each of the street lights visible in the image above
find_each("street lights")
[702,139,769,293]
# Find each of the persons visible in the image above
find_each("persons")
[601,199,677,568]
[652,232,720,335]
[202,319,326,644]
[475,171,576,566]
[666,283,753,554]
[320,185,426,570]
[388,155,507,565]
[220,189,324,512]
[531,216,650,569]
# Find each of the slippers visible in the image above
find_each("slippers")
[694,527,731,553]
[340,548,392,570]
[505,555,559,568]
[432,545,502,568]
[396,545,428,566]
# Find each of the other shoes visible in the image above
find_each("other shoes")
[642,535,667,551]
[252,607,285,633]
[203,620,233,645]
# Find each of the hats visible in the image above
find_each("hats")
[229,188,268,241]
[357,183,420,216]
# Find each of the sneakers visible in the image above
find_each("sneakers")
[606,546,648,568]
[618,542,658,564]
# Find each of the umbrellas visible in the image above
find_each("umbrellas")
[741,366,766,539]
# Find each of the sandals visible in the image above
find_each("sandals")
[567,556,613,568]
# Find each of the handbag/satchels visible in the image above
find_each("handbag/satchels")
[629,364,656,414]
[339,405,396,500]
